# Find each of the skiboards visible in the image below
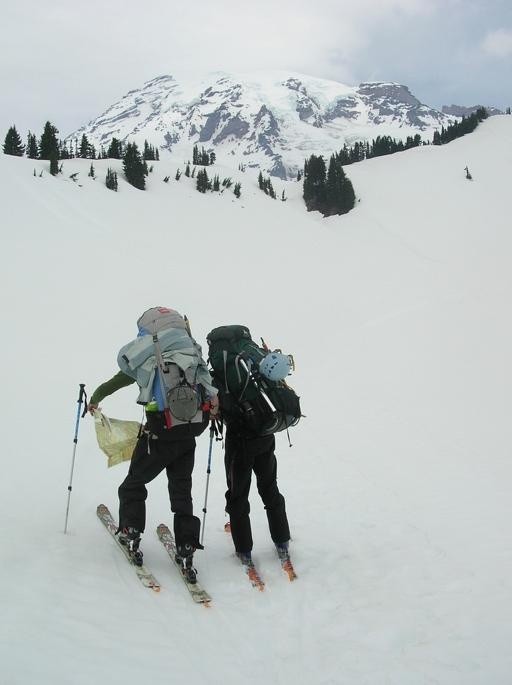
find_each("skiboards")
[97,504,212,605]
[224,522,297,593]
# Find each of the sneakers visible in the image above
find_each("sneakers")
[275,542,289,558]
[118,525,142,551]
[236,551,252,565]
[175,544,196,569]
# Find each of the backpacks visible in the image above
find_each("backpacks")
[208,325,300,437]
[133,306,211,442]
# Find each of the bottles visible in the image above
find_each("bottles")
[145,397,159,415]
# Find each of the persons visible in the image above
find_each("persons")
[86,307,208,573]
[208,325,292,565]
